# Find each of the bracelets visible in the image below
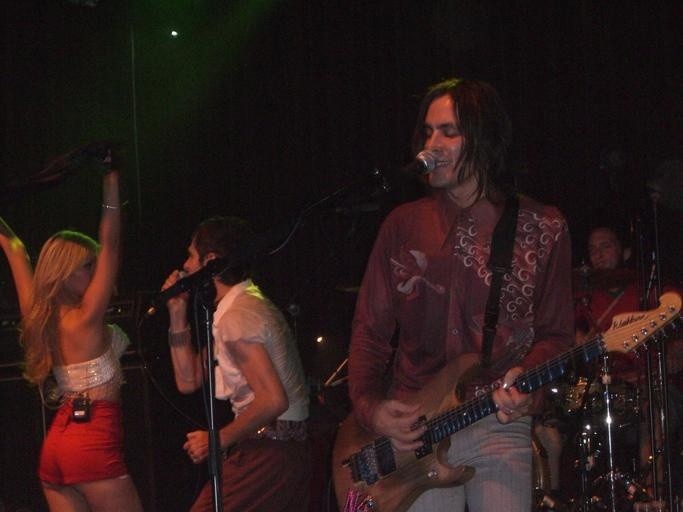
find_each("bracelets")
[165,321,192,346]
[100,200,120,209]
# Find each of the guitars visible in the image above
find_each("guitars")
[331,291,681,507]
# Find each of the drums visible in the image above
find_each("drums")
[544,374,644,435]
[530,414,612,511]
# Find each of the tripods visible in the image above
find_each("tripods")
[586,353,655,512]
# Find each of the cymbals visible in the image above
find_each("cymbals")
[571,265,634,291]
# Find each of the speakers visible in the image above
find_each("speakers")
[42,356,168,512]
[0,370,42,512]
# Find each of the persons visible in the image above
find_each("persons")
[348,77,574,512]
[537,220,681,512]
[0,146,145,511]
[161,216,324,511]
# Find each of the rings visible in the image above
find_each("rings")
[505,409,514,416]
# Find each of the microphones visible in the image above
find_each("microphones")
[371,149,437,207]
[146,251,231,308]
